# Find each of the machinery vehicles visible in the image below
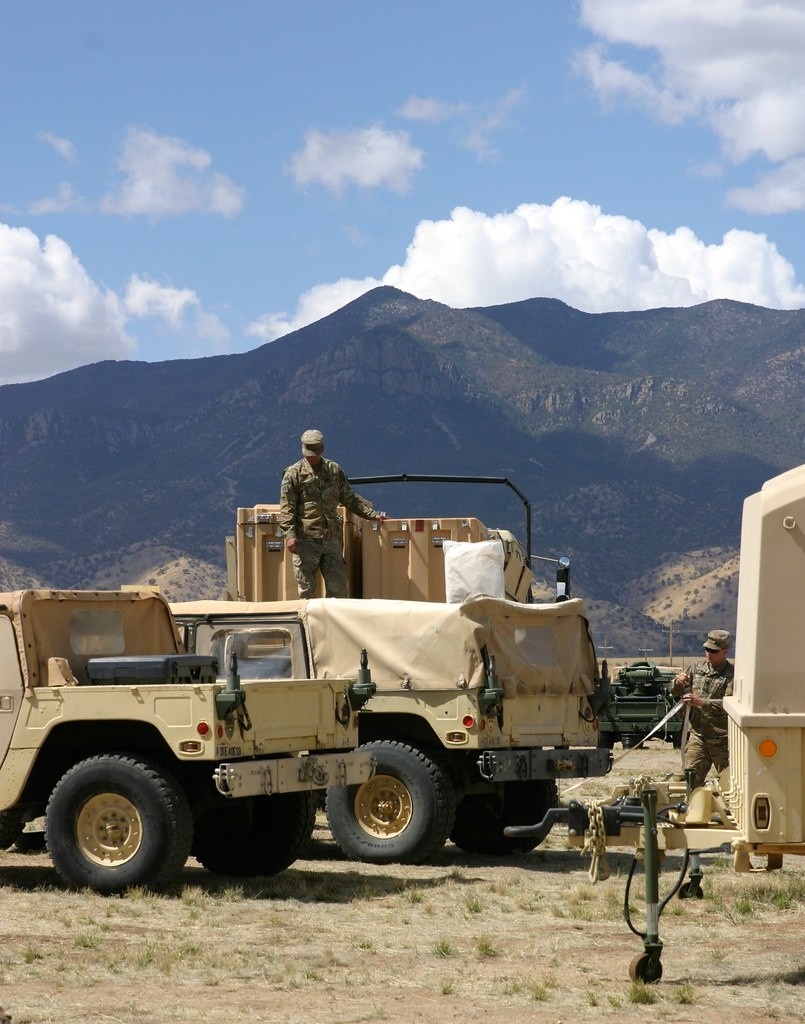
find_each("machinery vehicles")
[597,660,690,752]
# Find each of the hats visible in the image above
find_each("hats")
[703,630,731,650]
[301,429,324,456]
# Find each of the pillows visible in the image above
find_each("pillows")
[444,539,504,603]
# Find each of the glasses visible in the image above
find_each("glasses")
[704,647,721,654]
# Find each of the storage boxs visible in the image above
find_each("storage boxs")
[83,655,220,686]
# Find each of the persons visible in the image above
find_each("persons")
[669,630,734,791]
[278,429,392,599]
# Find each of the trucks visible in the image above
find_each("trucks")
[171,472,621,868]
[498,462,805,985]
[1,589,376,899]
